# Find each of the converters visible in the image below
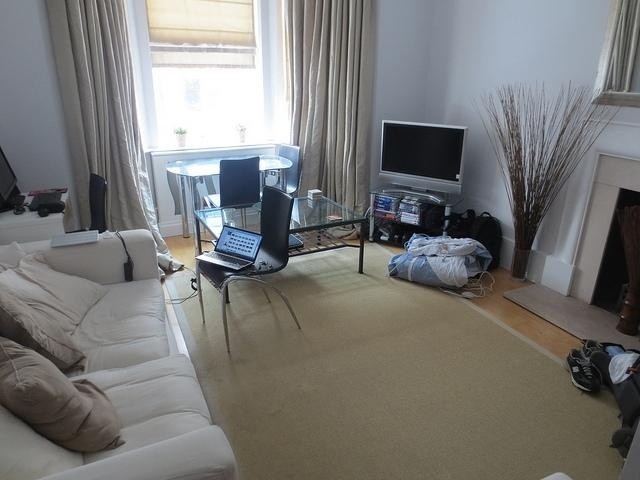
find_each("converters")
[125,263,132,282]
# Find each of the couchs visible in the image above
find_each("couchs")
[0,232,238,479]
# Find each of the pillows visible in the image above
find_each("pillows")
[1,250,127,452]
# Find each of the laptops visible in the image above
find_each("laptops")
[194,225,264,271]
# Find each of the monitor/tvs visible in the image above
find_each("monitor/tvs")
[0,146,25,212]
[379,120,468,203]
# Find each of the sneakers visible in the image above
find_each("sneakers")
[565,348,600,394]
[581,337,612,389]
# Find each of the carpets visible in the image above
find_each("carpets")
[168,241,632,479]
[503,284,640,357]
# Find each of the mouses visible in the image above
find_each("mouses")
[102,230,112,239]
[38,208,49,216]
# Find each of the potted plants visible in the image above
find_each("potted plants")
[174,127,187,148]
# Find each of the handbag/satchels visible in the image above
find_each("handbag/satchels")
[439,208,504,269]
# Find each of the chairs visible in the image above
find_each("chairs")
[67,173,107,236]
[197,185,301,358]
[202,143,303,229]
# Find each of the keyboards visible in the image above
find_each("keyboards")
[30,192,62,211]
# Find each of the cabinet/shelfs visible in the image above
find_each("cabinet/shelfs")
[368,184,465,244]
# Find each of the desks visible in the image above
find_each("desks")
[0,186,77,244]
[166,154,294,256]
